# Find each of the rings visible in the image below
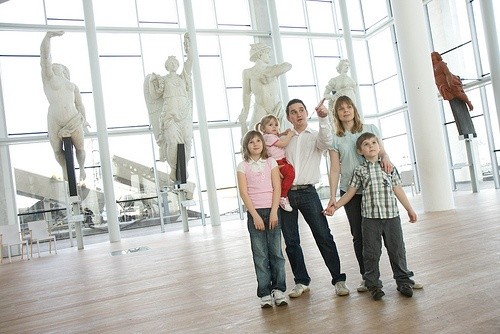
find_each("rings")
[321,110,324,111]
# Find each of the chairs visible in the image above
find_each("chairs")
[0,224,29,264]
[400,171,417,197]
[28,220,58,258]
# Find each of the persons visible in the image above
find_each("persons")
[323,59,365,126]
[237,41,292,131]
[84,207,94,227]
[236,131,289,308]
[279,98,349,297]
[431,52,478,139]
[145,33,195,182]
[254,116,296,212]
[322,95,424,291]
[40,30,90,182]
[322,134,418,298]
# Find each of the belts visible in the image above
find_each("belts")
[289,184,310,191]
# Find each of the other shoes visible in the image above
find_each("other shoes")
[396,284,413,297]
[357,283,368,292]
[334,281,349,296]
[412,282,424,289]
[260,295,273,308]
[288,284,310,298]
[271,289,288,306]
[279,196,293,212]
[372,288,385,301]
